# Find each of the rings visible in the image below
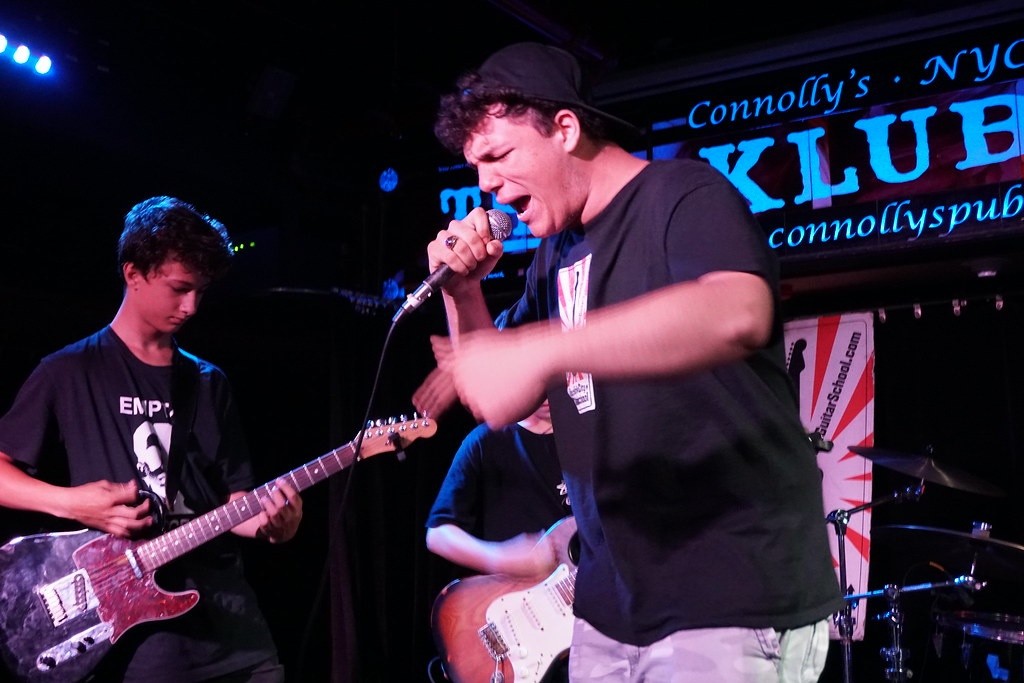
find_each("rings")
[445,235,458,249]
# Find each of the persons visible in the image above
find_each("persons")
[424,399,574,683]
[1,195,301,683]
[428,43,849,683]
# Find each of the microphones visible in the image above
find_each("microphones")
[392,209,512,325]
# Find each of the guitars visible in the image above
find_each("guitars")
[430,511,580,683]
[0,408,440,683]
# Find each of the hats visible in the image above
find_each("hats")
[477,42,638,129]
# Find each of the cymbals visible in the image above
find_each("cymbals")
[847,442,990,498]
[869,520,1024,560]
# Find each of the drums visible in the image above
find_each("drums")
[921,609,1024,683]
